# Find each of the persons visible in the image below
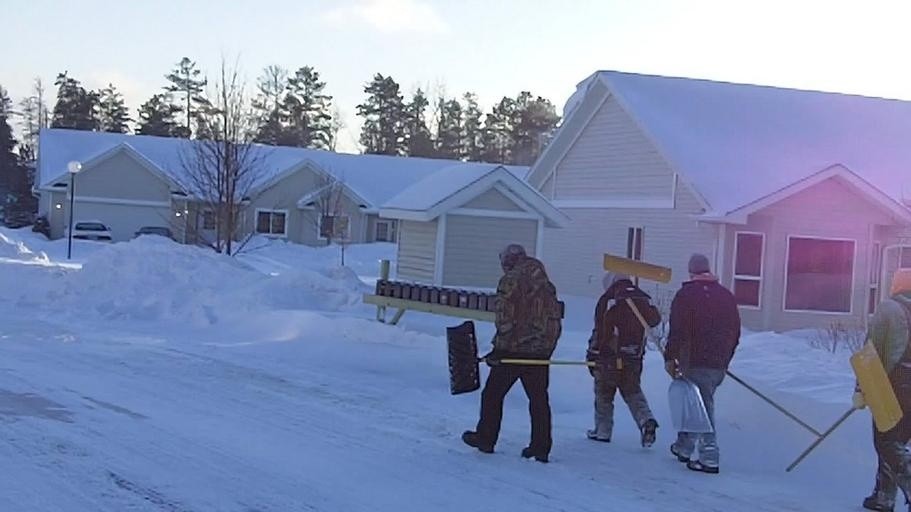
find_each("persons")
[585,271,660,448]
[850,268,910,512]
[664,253,740,474]
[460,243,561,463]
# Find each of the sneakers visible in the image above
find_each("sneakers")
[587,430,611,443]
[863,497,895,512]
[687,459,719,473]
[462,431,494,453]
[522,447,548,463]
[670,445,690,462]
[642,419,657,447]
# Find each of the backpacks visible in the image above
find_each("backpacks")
[511,270,564,351]
[599,287,647,359]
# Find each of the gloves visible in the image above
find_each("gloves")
[665,361,678,376]
[585,353,597,376]
[851,391,866,407]
[483,351,507,368]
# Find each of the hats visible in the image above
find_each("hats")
[600,270,632,290]
[501,244,525,269]
[890,268,910,296]
[688,254,710,274]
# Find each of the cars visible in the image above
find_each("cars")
[132,227,175,241]
[70,220,112,242]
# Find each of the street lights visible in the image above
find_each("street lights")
[65,161,80,260]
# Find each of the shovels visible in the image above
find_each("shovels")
[785,340,904,473]
[446,321,596,395]
[625,297,713,433]
[602,253,673,288]
